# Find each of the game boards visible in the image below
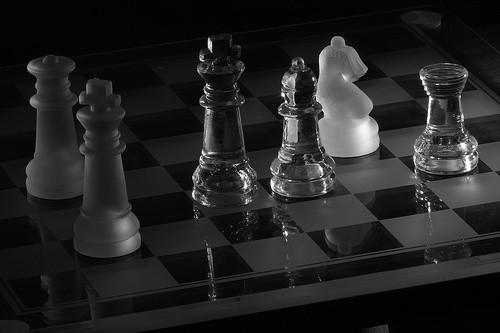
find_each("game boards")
[1,15,500,320]
